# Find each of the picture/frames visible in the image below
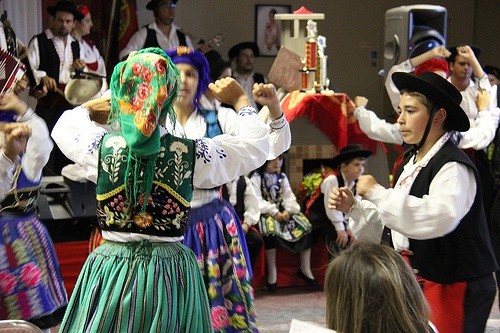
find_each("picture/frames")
[254,4,293,58]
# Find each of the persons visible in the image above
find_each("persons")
[119,0,194,62]
[204,49,233,82]
[240,151,319,288]
[329,71,499,333]
[264,9,281,55]
[353,39,500,196]
[28,2,109,176]
[308,141,370,261]
[0,22,48,123]
[228,40,269,114]
[0,90,68,324]
[324,240,440,333]
[162,47,292,333]
[50,47,271,333]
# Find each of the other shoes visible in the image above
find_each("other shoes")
[268,269,278,292]
[297,267,317,285]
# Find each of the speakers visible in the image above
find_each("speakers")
[383,4,447,84]
[36,178,100,240]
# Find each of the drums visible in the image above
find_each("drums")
[63,73,107,106]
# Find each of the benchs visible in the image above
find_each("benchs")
[276,236,329,287]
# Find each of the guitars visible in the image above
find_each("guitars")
[195,33,226,55]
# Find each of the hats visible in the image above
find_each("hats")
[391,69,471,133]
[45,0,85,19]
[329,144,374,166]
[228,41,261,60]
[145,0,178,9]
